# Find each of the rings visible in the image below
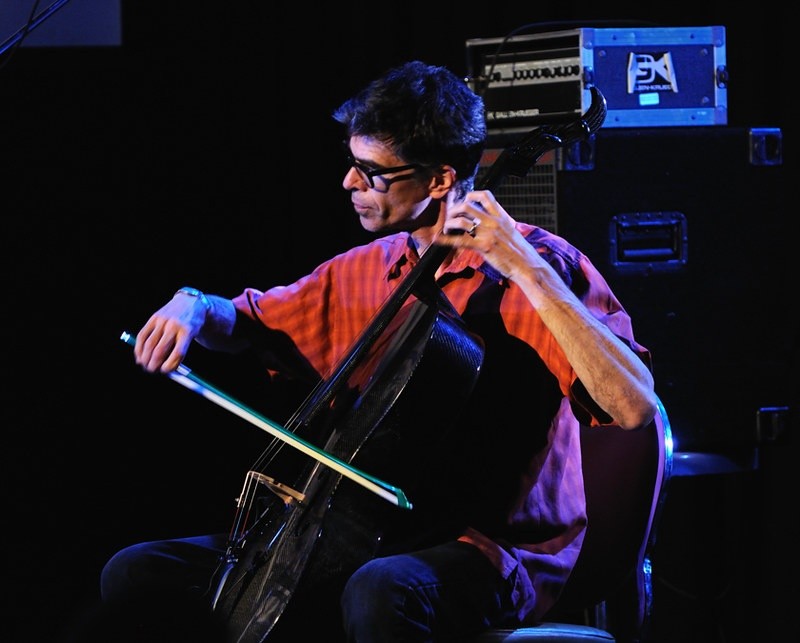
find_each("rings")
[467,222,477,235]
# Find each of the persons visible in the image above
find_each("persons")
[101,61,658,643]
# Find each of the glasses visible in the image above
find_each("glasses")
[340,140,427,189]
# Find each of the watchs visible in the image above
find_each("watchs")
[174,287,209,311]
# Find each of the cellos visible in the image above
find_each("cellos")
[199,82,611,643]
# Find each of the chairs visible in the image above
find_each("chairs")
[480,387,674,643]
